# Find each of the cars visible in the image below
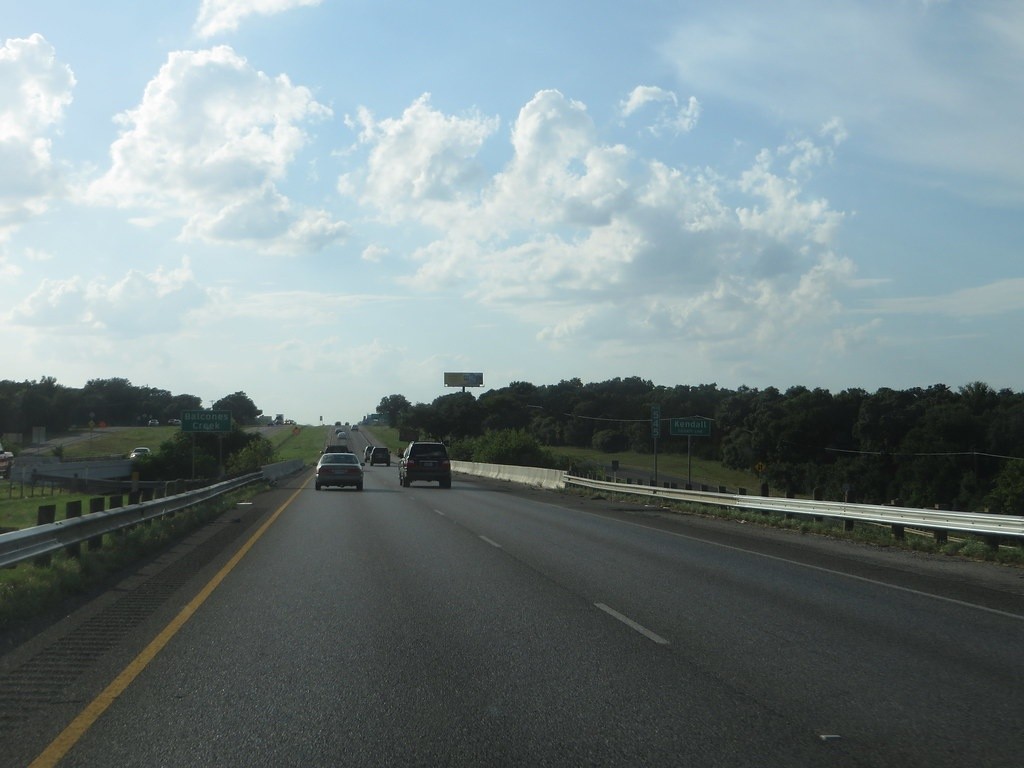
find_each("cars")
[313,453,366,491]
[168,419,176,426]
[363,446,375,462]
[369,447,391,467]
[335,421,341,426]
[149,419,159,426]
[130,447,153,459]
[335,428,342,433]
[338,432,346,439]
[0,443,14,479]
[174,420,182,425]
[345,422,350,426]
[320,445,354,454]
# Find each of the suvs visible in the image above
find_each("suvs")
[350,425,359,431]
[397,440,451,489]
